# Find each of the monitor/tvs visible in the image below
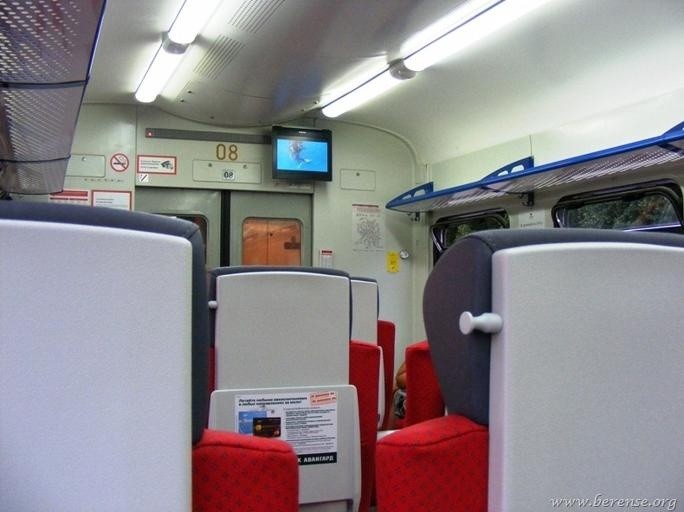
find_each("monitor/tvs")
[271,123,334,181]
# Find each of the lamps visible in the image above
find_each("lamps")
[135,1,218,103]
[322,0,551,118]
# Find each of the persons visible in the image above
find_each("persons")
[387,360,407,428]
[289,140,313,168]
